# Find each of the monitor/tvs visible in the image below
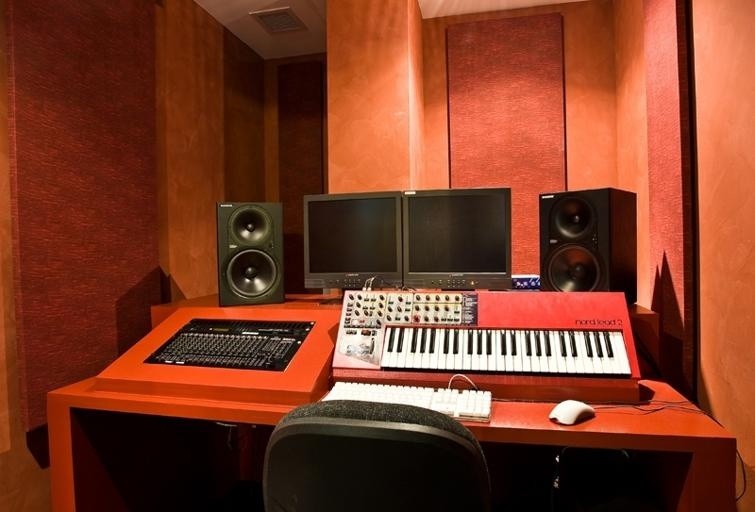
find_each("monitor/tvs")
[401,187,512,290]
[303,191,403,292]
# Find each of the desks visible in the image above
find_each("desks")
[45,375,736,512]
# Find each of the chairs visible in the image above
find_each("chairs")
[260,399,492,512]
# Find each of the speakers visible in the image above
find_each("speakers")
[216,200,285,306]
[538,187,637,306]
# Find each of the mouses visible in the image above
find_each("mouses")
[548,399,596,426]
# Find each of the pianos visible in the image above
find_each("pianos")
[331,290,642,404]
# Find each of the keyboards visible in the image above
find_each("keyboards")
[321,380,492,423]
